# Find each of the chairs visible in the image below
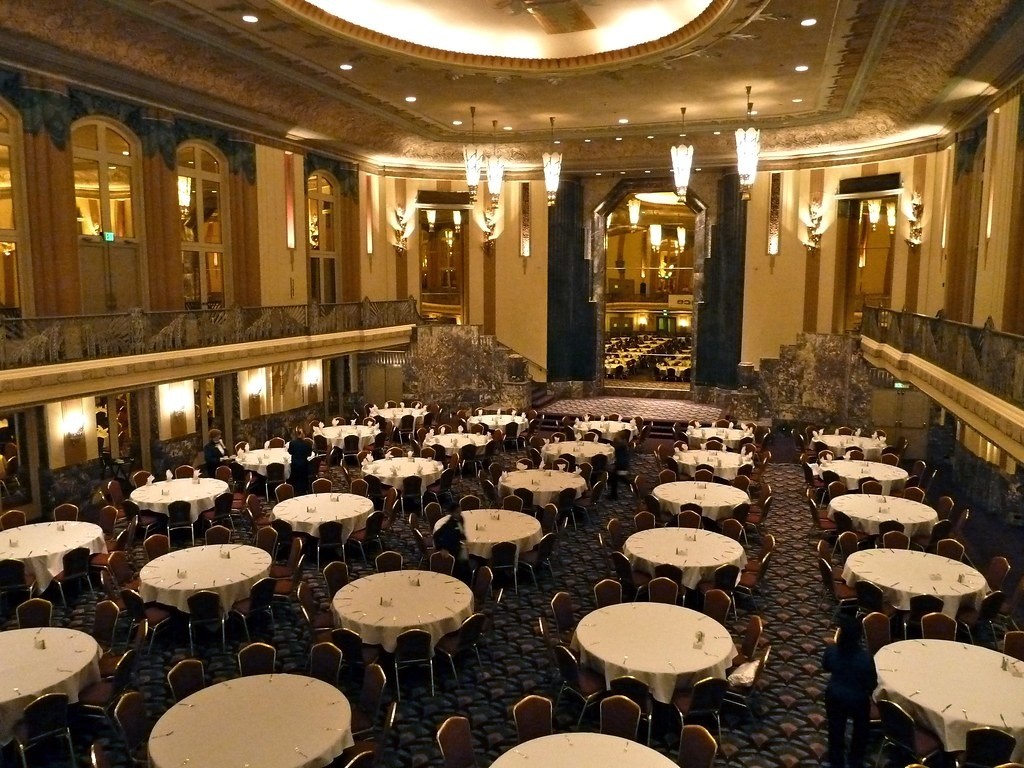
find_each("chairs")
[604,335,692,382]
[0,400,1024,768]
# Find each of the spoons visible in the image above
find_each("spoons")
[950,586,960,593]
[909,584,912,590]
[962,708,967,719]
[622,655,628,663]
[910,689,920,697]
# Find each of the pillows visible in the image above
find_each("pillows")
[728,659,760,686]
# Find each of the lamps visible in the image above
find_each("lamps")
[392,85,925,261]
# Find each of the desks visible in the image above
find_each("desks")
[840,548,988,621]
[496,469,590,509]
[313,422,382,453]
[367,406,429,429]
[430,508,544,561]
[421,433,494,456]
[827,492,939,538]
[268,492,375,547]
[622,527,749,591]
[0,627,103,749]
[234,447,318,479]
[137,544,273,632]
[540,440,616,467]
[685,426,756,451]
[465,414,530,439]
[128,477,230,525]
[568,601,739,704]
[148,674,355,768]
[873,638,1024,763]
[488,732,682,768]
[809,433,883,463]
[672,450,756,481]
[818,459,908,496]
[361,457,443,498]
[0,520,108,598]
[572,420,639,443]
[330,569,474,659]
[652,480,750,522]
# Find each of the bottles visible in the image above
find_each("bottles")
[1001,656,1009,671]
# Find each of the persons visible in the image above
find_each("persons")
[205,429,230,478]
[643,334,648,341]
[434,501,473,588]
[96,412,108,446]
[287,428,312,495]
[0,454,8,480]
[822,617,878,768]
[602,430,630,501]
[612,338,630,352]
[650,336,653,340]
[636,335,644,344]
[629,337,639,348]
[607,346,612,352]
[667,338,680,354]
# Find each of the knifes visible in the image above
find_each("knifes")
[344,611,361,615]
[933,586,938,594]
[168,581,180,589]
[1000,714,1007,728]
[858,572,872,573]
[891,582,899,587]
[962,584,974,590]
[375,617,384,625]
[213,579,215,586]
[28,551,32,557]
[941,704,952,713]
[241,573,249,577]
